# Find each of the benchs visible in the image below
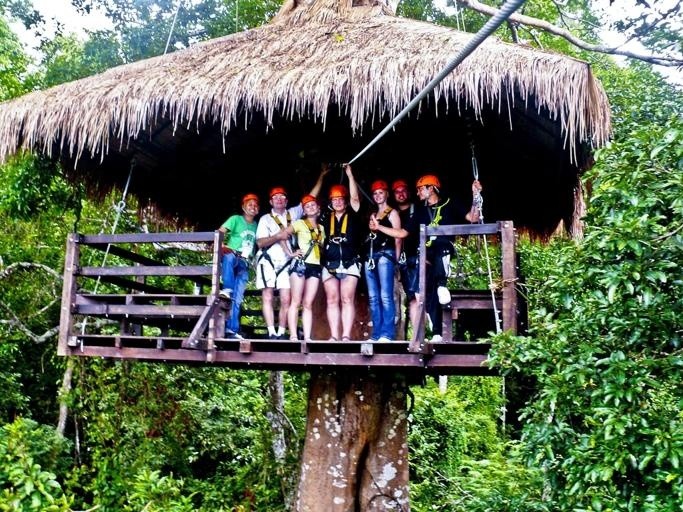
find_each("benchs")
[440,288,505,343]
[69,292,224,339]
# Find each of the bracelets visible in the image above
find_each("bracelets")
[287,253,292,259]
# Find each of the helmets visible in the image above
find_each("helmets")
[415,174,441,189]
[269,185,287,200]
[300,193,317,208]
[370,180,388,192]
[242,193,260,203]
[390,179,407,190]
[328,183,348,199]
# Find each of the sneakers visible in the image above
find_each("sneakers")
[435,286,453,305]
[227,330,444,342]
[218,289,230,300]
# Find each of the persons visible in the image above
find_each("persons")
[367,180,425,344]
[366,175,483,342]
[216,194,260,339]
[278,194,327,342]
[320,162,362,342]
[364,178,402,342]
[254,161,330,341]
